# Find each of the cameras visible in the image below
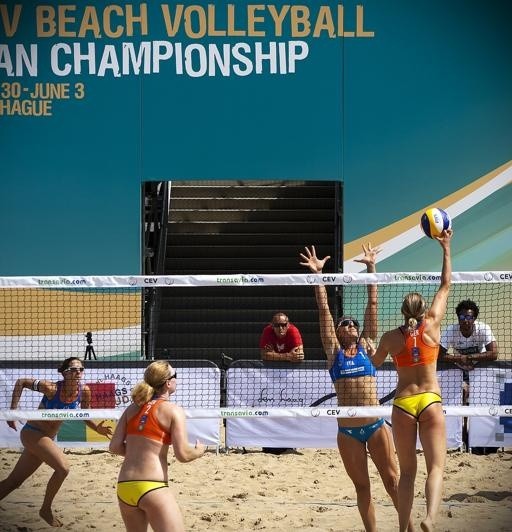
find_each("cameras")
[86,333,92,338]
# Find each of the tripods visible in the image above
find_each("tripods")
[84,338,97,360]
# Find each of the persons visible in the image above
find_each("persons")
[438,299,498,369]
[109,360,207,532]
[0,357,113,527]
[299,242,415,532]
[366,228,454,532]
[259,312,304,363]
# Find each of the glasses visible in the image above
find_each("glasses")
[336,319,359,331]
[459,314,475,320]
[65,367,84,371]
[161,371,177,387]
[273,322,288,327]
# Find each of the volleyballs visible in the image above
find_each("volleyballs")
[421,207,452,239]
